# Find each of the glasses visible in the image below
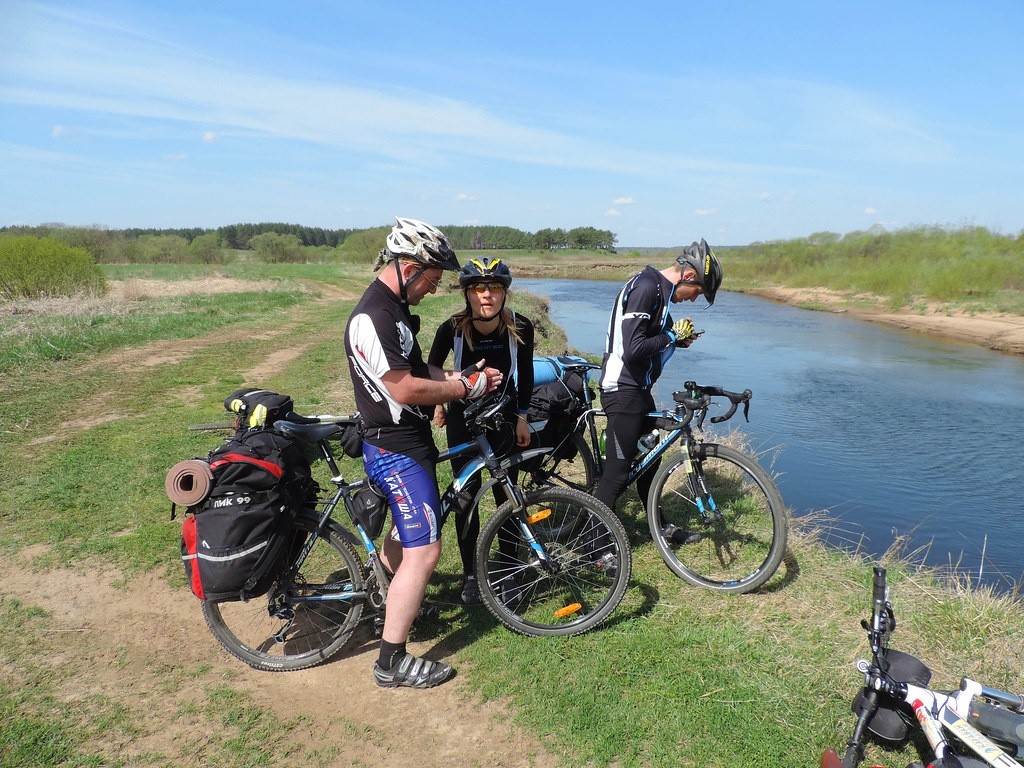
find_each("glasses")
[403,263,441,290]
[467,281,505,294]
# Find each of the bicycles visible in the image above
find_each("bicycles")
[186,362,632,674]
[510,357,789,595]
[838,565,1024,768]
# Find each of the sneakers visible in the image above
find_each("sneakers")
[374,601,440,625]
[650,523,702,543]
[589,552,618,578]
[372,653,453,689]
[461,571,482,604]
[500,574,522,606]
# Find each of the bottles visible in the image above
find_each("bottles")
[630,428,661,468]
[598,424,608,457]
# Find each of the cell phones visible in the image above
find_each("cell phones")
[692,329,705,335]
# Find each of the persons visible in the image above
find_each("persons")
[428,256,534,606]
[343,218,504,690]
[585,237,723,577]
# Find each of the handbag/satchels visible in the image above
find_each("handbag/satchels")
[340,423,363,459]
[516,370,596,472]
[180,388,320,603]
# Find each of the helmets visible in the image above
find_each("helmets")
[387,215,461,272]
[459,256,512,290]
[683,238,723,306]
